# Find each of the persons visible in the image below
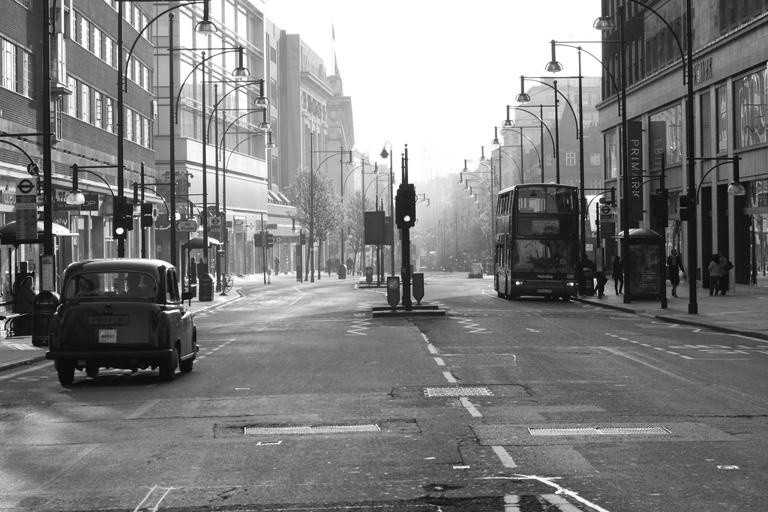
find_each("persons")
[124,273,145,294]
[667,248,684,298]
[16,276,37,314]
[327,257,354,275]
[708,251,733,296]
[274,256,280,275]
[612,256,623,295]
[187,257,207,280]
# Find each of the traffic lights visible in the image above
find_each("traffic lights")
[397,182,417,228]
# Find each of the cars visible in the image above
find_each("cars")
[43,257,198,391]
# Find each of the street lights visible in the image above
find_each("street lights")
[307,131,396,282]
[379,145,395,277]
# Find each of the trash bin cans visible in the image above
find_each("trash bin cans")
[33,290,61,346]
[579,268,594,296]
[339,264,347,279]
[199,273,214,301]
